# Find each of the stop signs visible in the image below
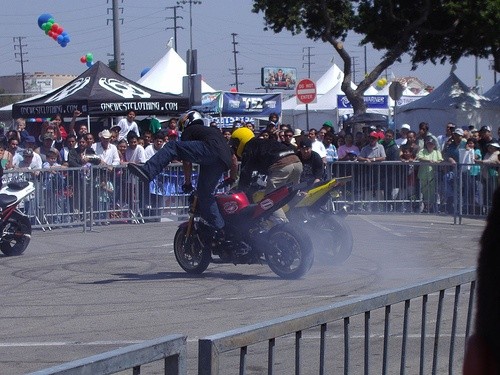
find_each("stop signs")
[295,79,318,105]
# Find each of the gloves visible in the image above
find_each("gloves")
[182,184,193,194]
[218,178,235,189]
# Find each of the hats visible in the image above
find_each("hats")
[481,126,491,133]
[110,125,122,132]
[368,131,379,139]
[377,132,384,140]
[322,120,334,127]
[452,128,464,136]
[23,148,34,156]
[168,130,178,137]
[300,138,312,148]
[294,128,302,138]
[266,121,276,127]
[101,130,112,139]
[401,124,412,131]
[44,133,54,141]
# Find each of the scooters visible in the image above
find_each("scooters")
[0,176,36,256]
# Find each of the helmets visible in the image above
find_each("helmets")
[228,128,256,158]
[177,109,204,133]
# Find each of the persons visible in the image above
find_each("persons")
[18,148,39,217]
[295,138,323,189]
[3,138,24,169]
[42,151,68,189]
[230,126,304,224]
[209,113,500,213]
[0,109,179,229]
[461,180,500,375]
[276,69,286,87]
[128,110,238,246]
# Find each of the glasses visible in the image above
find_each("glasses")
[420,128,425,131]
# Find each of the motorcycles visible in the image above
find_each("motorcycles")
[225,171,354,266]
[173,176,315,280]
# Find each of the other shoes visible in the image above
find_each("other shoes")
[127,162,152,183]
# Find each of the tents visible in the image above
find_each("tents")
[278,62,378,135]
[397,73,500,133]
[12,59,190,133]
[135,48,216,96]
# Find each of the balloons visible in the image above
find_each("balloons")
[87,62,93,68]
[80,56,86,63]
[377,80,384,87]
[38,13,71,48]
[382,78,387,84]
[87,56,93,62]
[87,53,92,57]
